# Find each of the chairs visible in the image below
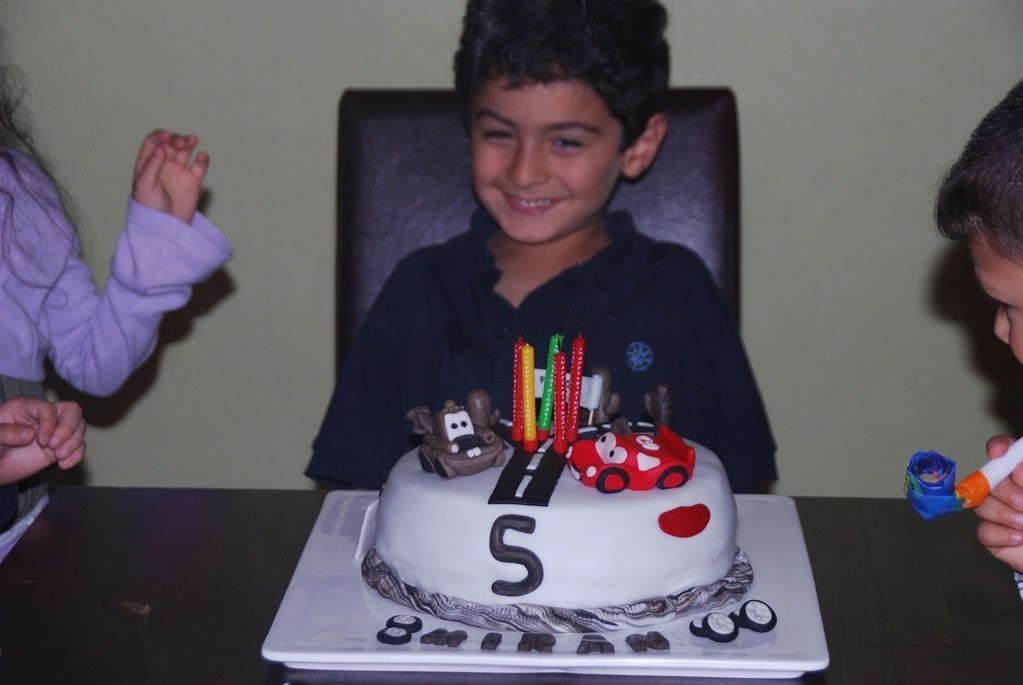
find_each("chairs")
[333,87,740,382]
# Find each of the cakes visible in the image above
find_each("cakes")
[359,330,754,635]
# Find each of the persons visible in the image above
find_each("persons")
[303,0,780,497]
[933,80,1023,572]
[0,60,233,551]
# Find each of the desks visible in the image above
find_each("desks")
[0,484,1023,685]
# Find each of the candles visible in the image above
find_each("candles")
[512,330,586,456]
